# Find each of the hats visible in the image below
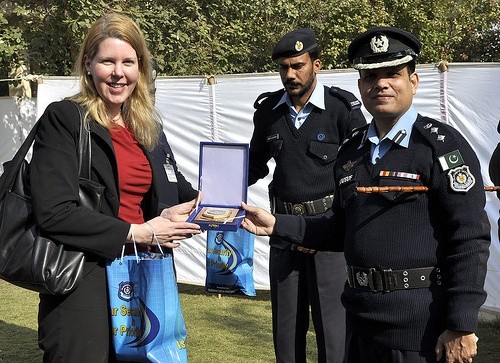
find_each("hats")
[271,27,318,62]
[347,26,423,70]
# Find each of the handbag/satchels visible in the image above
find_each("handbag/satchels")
[1,156,106,298]
[105,223,188,363]
[203,211,257,298]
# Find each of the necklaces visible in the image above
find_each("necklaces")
[111,114,122,122]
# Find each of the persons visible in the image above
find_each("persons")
[30,13,225,363]
[217,27,367,363]
[241,27,491,363]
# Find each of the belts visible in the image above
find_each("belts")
[269,194,336,217]
[344,264,439,294]
